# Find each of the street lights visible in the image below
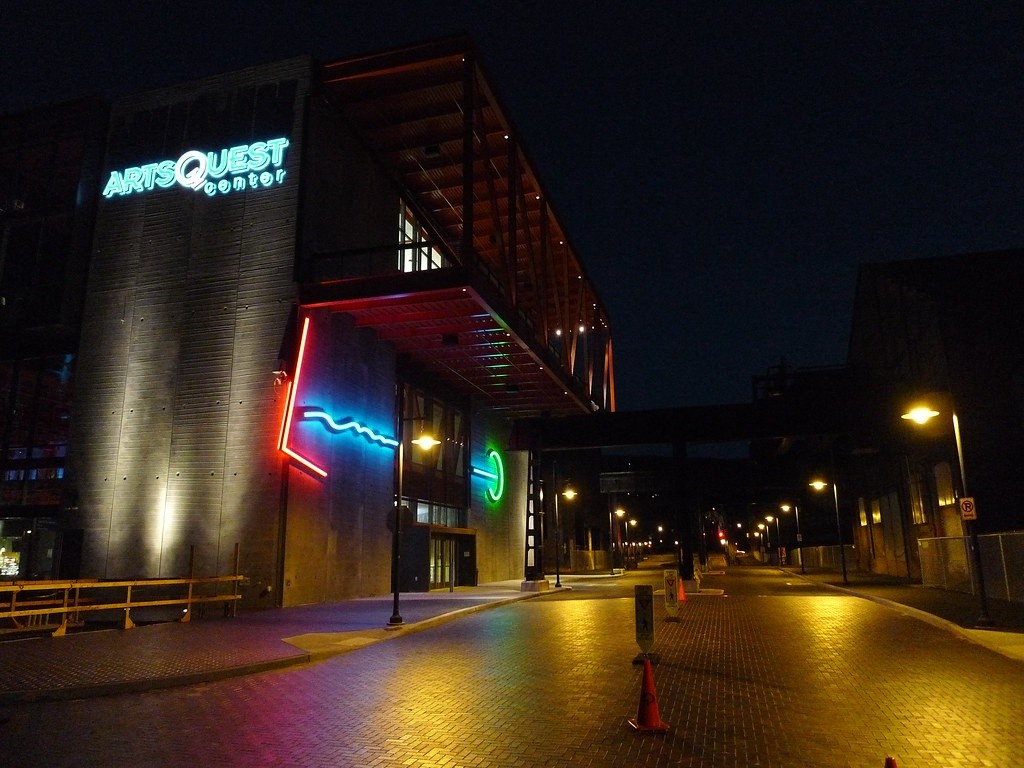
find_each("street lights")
[781,505,806,574]
[754,532,764,564]
[758,524,771,558]
[766,515,782,566]
[901,390,996,630]
[553,461,579,588]
[625,519,637,562]
[809,480,848,585]
[607,492,626,575]
[389,383,443,623]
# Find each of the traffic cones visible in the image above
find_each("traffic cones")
[678,578,686,601]
[626,659,670,733]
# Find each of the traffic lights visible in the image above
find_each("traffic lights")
[718,532,724,538]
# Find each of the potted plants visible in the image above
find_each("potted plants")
[520,568,549,592]
